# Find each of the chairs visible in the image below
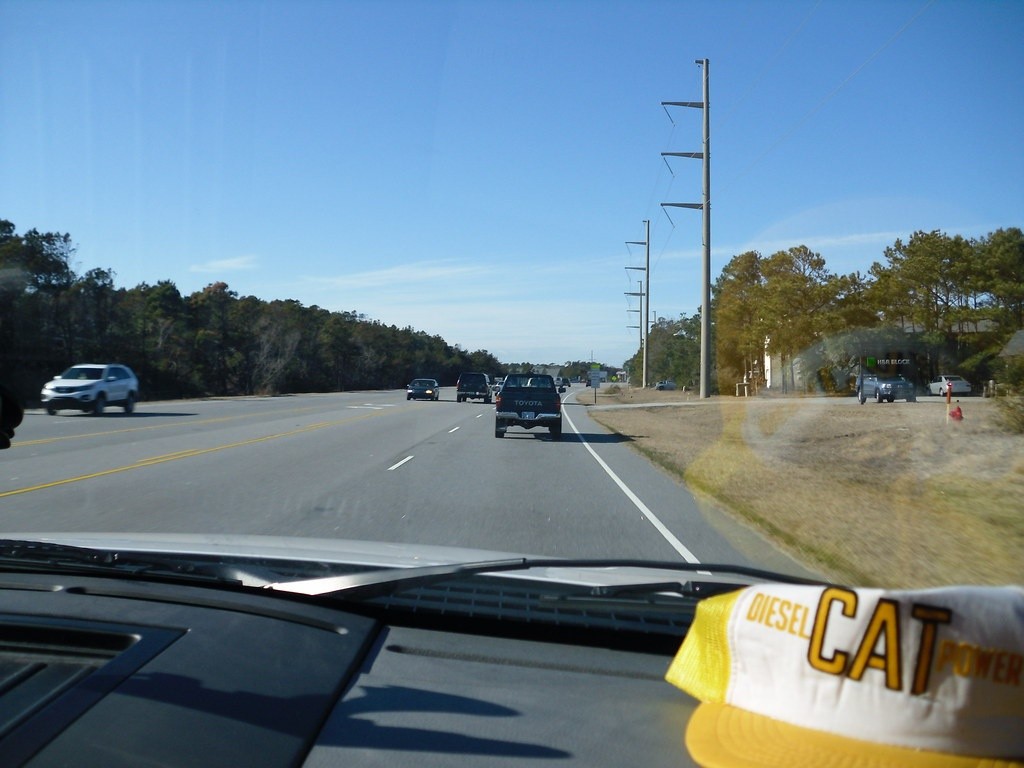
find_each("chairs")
[77,372,87,379]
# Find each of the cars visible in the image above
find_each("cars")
[526,378,550,388]
[627,377,631,384]
[492,381,511,391]
[655,380,676,390]
[562,379,572,387]
[586,380,591,387]
[744,371,760,385]
[855,369,916,403]
[40,364,139,416]
[924,375,971,396]
[456,372,493,403]
[406,379,439,401]
[553,377,564,386]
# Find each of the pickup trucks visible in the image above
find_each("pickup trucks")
[494,373,567,438]
